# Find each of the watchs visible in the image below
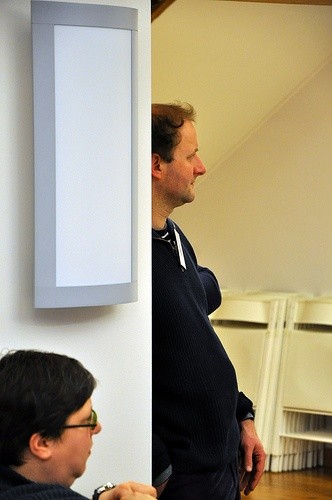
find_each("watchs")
[92,481,117,500]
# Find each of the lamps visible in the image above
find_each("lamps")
[31,0,141,309]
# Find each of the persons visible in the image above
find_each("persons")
[151,103,267,500]
[0,349,157,500]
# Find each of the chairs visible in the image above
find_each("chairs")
[208,289,332,473]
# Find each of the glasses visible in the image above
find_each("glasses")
[40,409,97,437]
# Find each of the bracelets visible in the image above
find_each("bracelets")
[242,412,254,421]
